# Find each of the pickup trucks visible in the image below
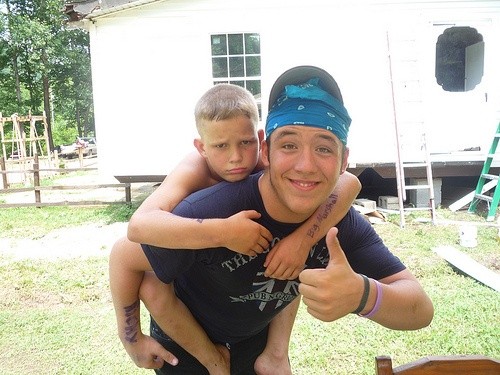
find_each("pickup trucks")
[60,137,97,160]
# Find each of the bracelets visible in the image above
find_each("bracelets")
[357,279,383,320]
[352,273,370,314]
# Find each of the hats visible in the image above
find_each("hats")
[268,65,343,112]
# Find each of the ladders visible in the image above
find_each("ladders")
[468,120,500,220]
[384,25,440,229]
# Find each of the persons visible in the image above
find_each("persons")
[127,83,362,375]
[108,65,434,375]
